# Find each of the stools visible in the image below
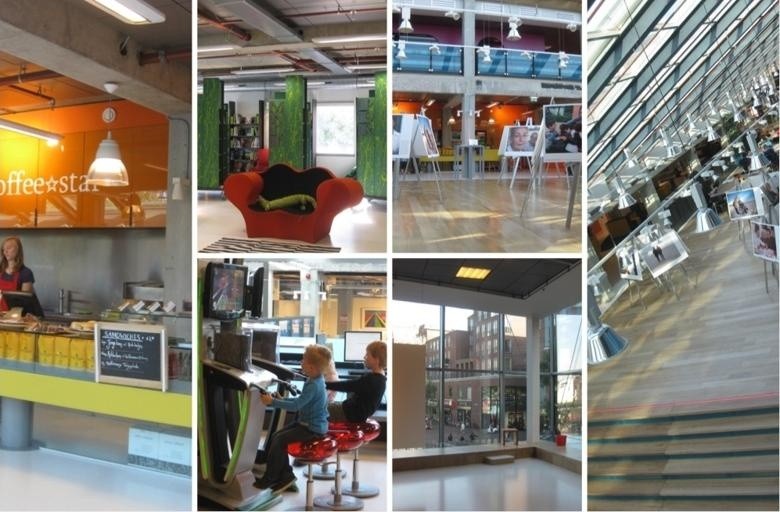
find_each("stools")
[289,420,382,512]
[502,428,519,447]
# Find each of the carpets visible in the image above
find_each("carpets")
[199,237,341,254]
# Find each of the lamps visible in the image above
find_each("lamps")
[585,0,779,368]
[393,0,581,71]
[85,82,129,188]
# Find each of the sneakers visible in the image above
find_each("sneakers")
[253,471,297,494]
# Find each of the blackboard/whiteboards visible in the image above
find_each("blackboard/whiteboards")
[94,321,168,393]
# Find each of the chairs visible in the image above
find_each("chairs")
[222,163,365,243]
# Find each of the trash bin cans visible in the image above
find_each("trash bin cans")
[0,396,33,451]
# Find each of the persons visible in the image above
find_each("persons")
[0,237,44,318]
[325,342,387,422]
[253,344,332,496]
[508,127,538,151]
[754,224,776,256]
[652,245,666,262]
[545,119,582,153]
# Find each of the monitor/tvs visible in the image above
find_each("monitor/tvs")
[1,290,44,320]
[344,331,382,375]
[251,267,264,318]
[203,262,248,320]
